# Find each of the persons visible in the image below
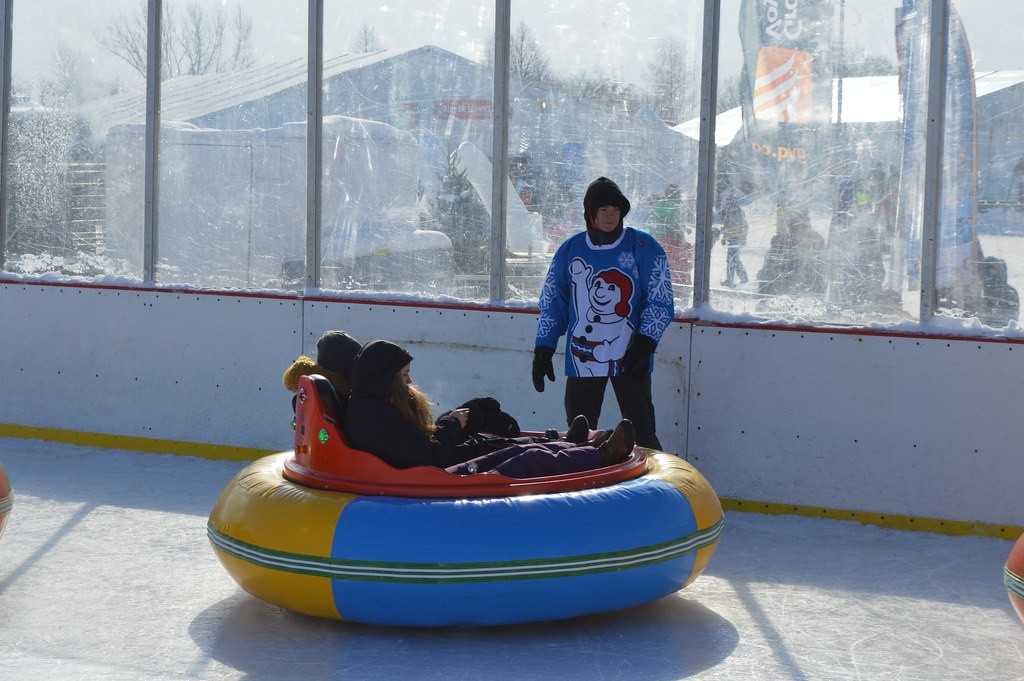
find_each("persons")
[531,177,675,452]
[283,329,638,477]
[645,161,1019,325]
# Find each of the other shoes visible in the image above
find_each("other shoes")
[738,269,748,282]
[721,280,735,288]
[563,415,588,444]
[545,427,558,440]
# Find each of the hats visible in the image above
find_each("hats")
[353,340,414,377]
[316,330,362,369]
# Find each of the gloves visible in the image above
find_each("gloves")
[456,396,520,437]
[623,333,655,382]
[532,349,555,392]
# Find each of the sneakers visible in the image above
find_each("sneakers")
[592,429,613,447]
[597,419,635,464]
